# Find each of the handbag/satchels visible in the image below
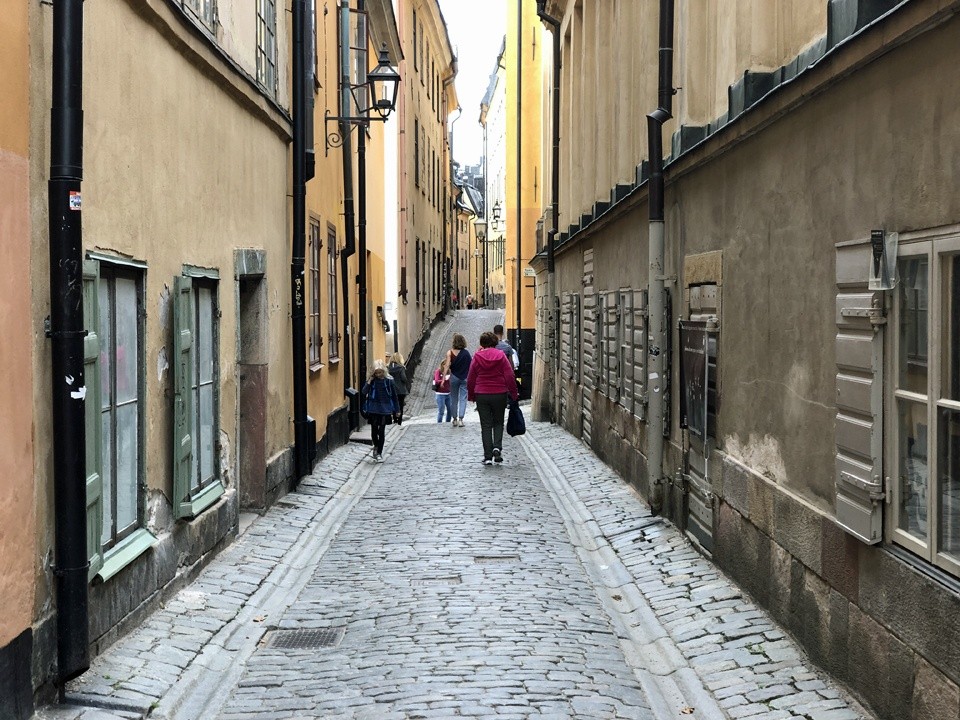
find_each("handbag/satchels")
[432,379,440,392]
[506,399,526,437]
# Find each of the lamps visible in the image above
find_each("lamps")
[493,199,506,222]
[474,207,505,244]
[323,44,401,157]
[492,220,507,232]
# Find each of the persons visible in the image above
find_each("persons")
[467,333,518,465]
[432,325,519,427]
[387,351,408,425]
[361,360,402,464]
[465,292,474,310]
[452,292,458,311]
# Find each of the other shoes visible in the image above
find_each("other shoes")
[458,418,466,427]
[452,419,458,426]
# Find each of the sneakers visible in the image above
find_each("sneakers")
[481,458,492,465]
[492,448,501,460]
[372,453,384,463]
